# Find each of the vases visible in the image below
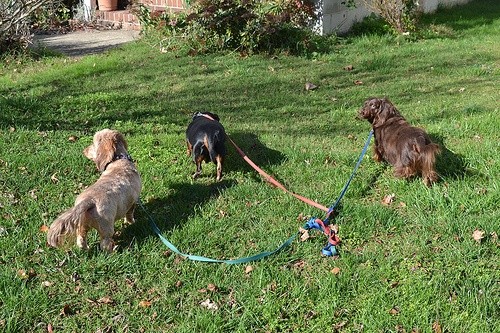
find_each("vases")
[98,0,117,11]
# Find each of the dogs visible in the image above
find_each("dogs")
[46,129,145,253]
[185,109,229,182]
[358,95,443,188]
